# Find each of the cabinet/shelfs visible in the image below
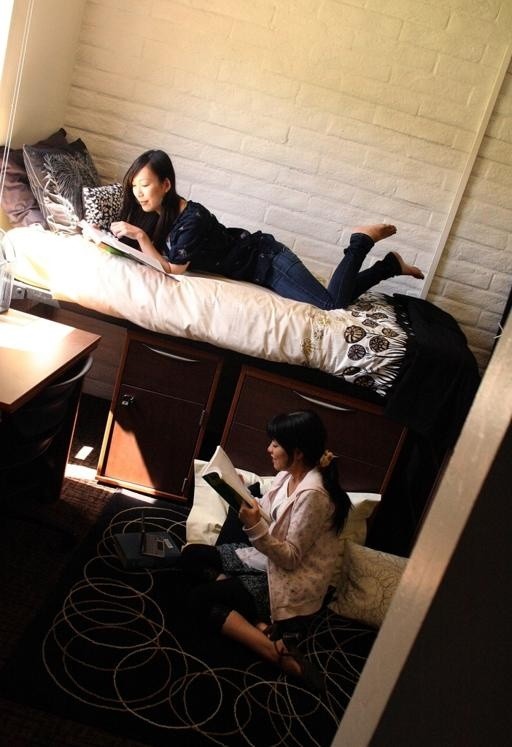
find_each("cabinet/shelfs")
[95,327,224,500]
[0,305,103,473]
[217,364,408,495]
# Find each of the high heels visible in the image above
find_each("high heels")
[261,623,282,642]
[277,631,312,684]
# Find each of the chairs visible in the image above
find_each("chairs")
[0,352,94,517]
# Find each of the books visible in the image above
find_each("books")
[77,219,167,275]
[199,442,274,526]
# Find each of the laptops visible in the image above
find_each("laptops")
[140,512,166,558]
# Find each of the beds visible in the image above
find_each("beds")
[0,223,478,431]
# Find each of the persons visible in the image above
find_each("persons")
[90,148,427,310]
[171,406,355,684]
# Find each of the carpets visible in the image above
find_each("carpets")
[0,495,380,747]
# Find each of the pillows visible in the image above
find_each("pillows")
[23,137,101,235]
[328,540,408,627]
[81,183,125,232]
[0,128,67,229]
[180,459,381,588]
